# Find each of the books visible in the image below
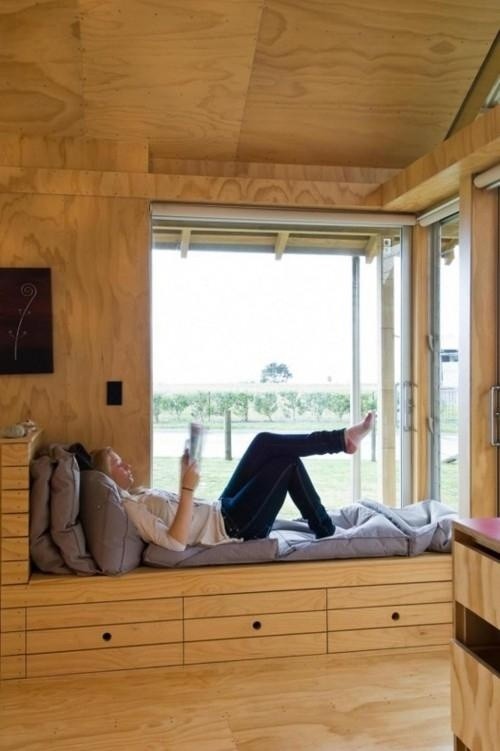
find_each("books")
[188,419,204,468]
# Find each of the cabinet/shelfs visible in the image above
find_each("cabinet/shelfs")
[450,519,500,751]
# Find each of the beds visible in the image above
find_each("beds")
[1,428,453,681]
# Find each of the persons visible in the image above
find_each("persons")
[88,409,381,555]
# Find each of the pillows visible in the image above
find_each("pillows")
[79,470,144,574]
[49,444,96,576]
[28,444,69,575]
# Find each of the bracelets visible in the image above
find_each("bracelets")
[182,488,193,491]
